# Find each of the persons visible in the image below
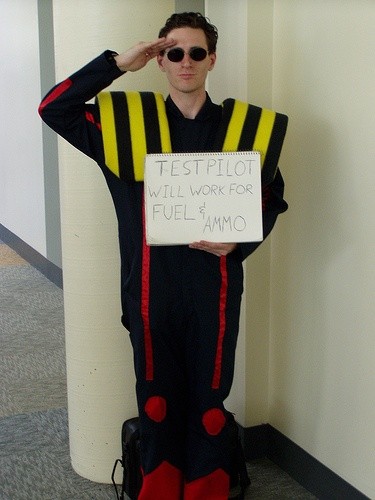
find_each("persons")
[39,9,290,419]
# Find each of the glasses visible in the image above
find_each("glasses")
[162,47,209,63]
